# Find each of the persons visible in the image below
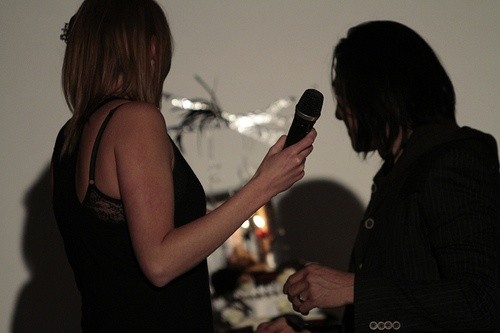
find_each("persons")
[51,0,317,333]
[283,21,500,333]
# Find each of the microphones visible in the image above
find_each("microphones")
[281,88,324,153]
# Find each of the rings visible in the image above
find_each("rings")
[297,293,305,303]
